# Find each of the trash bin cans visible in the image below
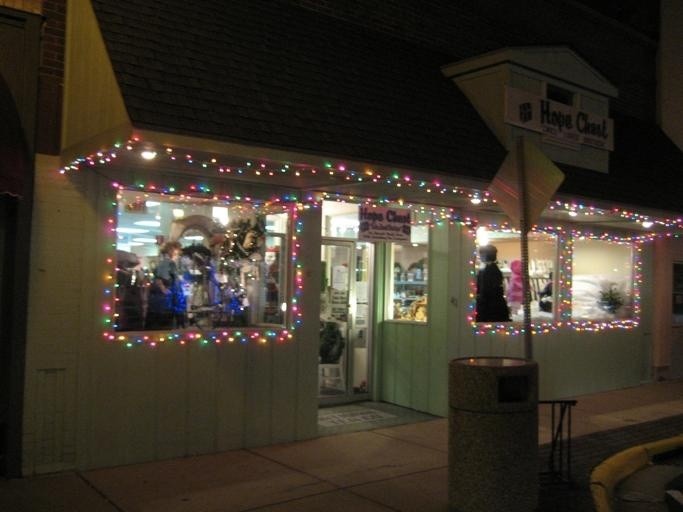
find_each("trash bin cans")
[448,356,539,512]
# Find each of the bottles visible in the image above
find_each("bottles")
[392,267,430,284]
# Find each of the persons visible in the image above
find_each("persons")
[508,261,524,315]
[478,246,508,322]
[145,241,182,329]
[538,273,552,311]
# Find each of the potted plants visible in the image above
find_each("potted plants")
[595,286,623,314]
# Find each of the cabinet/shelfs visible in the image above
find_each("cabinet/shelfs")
[393,278,427,310]
